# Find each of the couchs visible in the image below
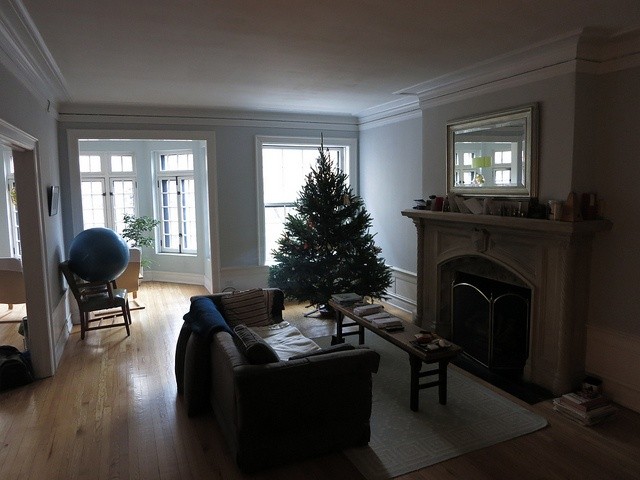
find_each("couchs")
[188,286,382,469]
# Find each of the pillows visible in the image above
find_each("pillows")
[232,322,281,364]
[289,342,356,361]
[221,288,271,325]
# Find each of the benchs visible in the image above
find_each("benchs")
[328,292,462,412]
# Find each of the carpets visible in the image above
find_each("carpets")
[309,328,549,480]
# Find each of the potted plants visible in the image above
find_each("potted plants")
[264,130,395,319]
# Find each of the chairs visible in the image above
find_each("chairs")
[115,246,141,299]
[57,259,133,341]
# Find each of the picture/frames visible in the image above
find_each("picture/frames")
[49,185,60,217]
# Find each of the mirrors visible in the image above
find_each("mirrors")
[444,102,539,202]
